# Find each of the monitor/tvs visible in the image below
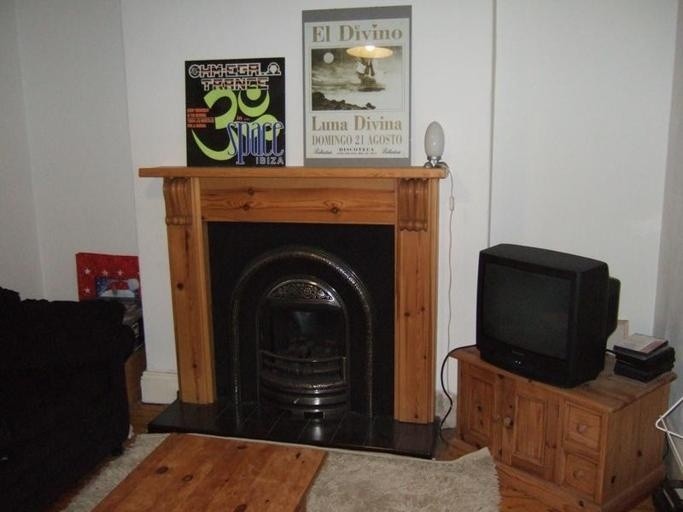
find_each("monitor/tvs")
[476,243,620,388]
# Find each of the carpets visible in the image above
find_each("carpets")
[59,431,503,511]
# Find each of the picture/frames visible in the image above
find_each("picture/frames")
[299,3,414,171]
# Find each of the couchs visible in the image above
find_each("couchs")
[0,282,137,510]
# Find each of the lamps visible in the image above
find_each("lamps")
[418,118,449,170]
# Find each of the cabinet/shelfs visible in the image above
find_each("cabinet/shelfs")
[438,342,682,511]
